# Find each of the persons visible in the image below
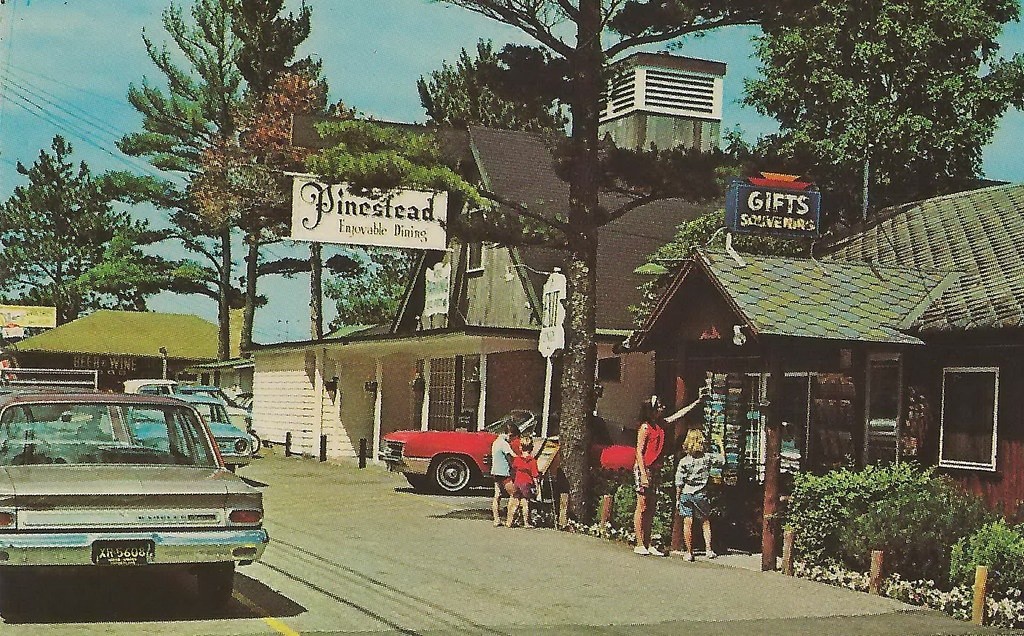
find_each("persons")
[491,424,520,528]
[674,427,726,563]
[632,386,710,558]
[505,437,540,530]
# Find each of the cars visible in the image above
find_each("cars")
[0,386,269,608]
[129,384,252,472]
[377,404,643,497]
